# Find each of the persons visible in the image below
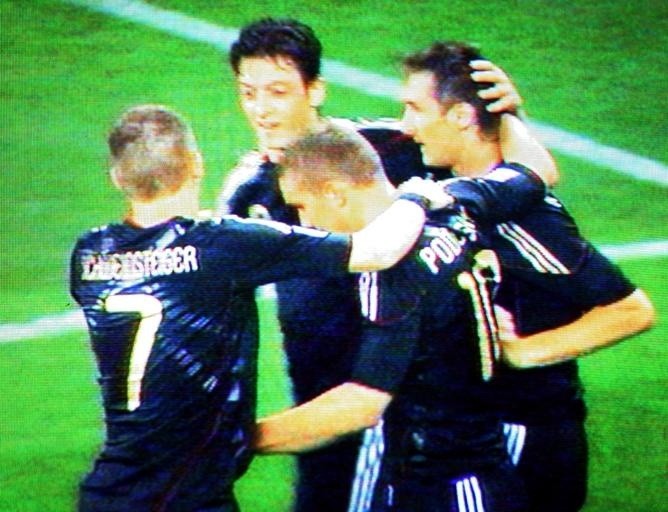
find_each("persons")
[403,35,658,512]
[66,102,448,509]
[208,16,526,511]
[240,114,557,511]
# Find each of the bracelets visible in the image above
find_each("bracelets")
[398,193,432,216]
